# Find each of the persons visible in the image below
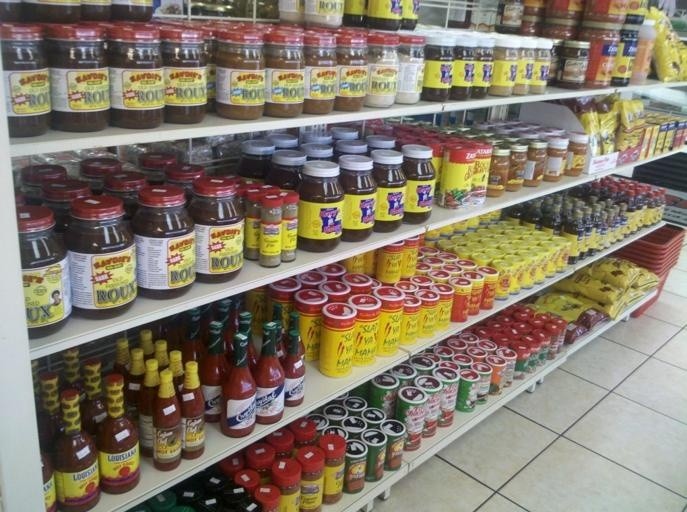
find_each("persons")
[52,290,61,305]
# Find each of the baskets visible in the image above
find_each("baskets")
[613,225,686,318]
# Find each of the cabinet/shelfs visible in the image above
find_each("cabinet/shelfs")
[0,74,687,510]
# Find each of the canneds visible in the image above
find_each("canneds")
[12,119,592,340]
[31,208,571,512]
[1,0,648,138]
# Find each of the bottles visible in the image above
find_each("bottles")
[220,332,258,438]
[503,176,668,266]
[139,329,155,360]
[246,445,278,484]
[165,349,185,402]
[127,347,144,418]
[297,445,324,512]
[254,321,286,425]
[632,20,658,81]
[62,348,82,393]
[152,339,170,372]
[201,320,227,425]
[183,308,203,363]
[113,337,130,372]
[35,373,63,450]
[98,373,142,492]
[272,457,303,512]
[54,390,100,512]
[320,434,347,504]
[263,429,297,458]
[290,417,318,446]
[283,313,306,408]
[179,361,205,460]
[80,355,107,434]
[151,369,183,470]
[235,311,258,371]
[139,358,159,458]
